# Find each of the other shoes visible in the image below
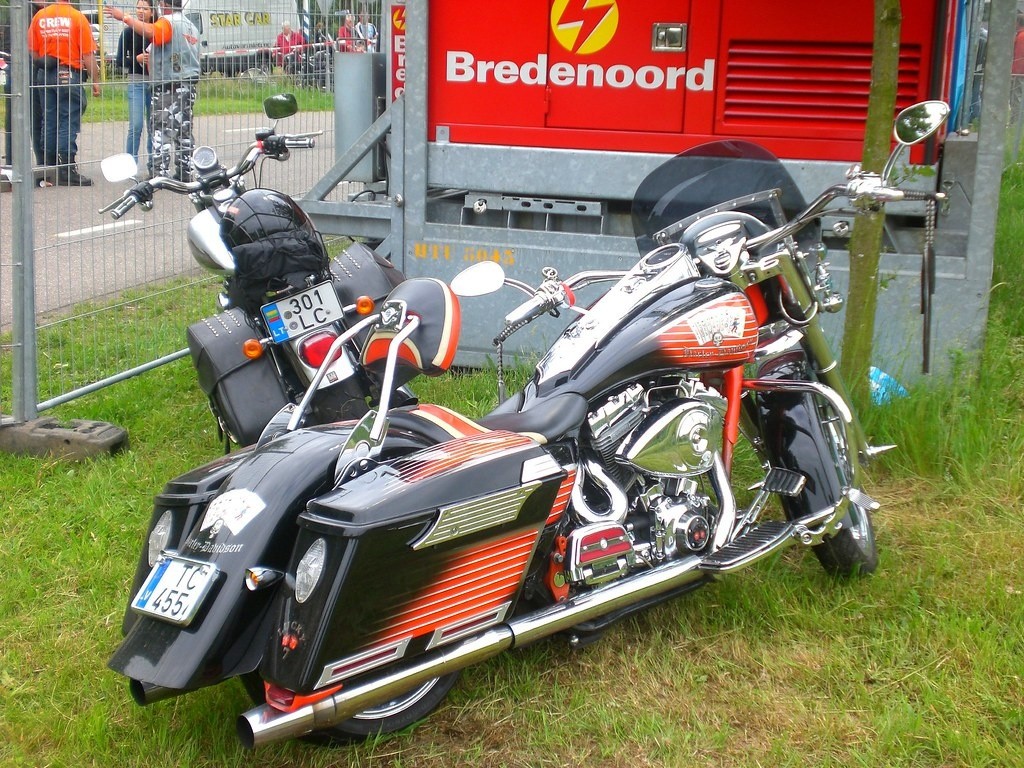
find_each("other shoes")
[51,170,94,186]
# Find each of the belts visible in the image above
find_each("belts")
[152,84,180,90]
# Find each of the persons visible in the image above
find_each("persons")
[103,0,201,182]
[271,12,380,75]
[116,0,158,180]
[4,0,100,186]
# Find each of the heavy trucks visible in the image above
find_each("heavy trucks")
[80,1,305,74]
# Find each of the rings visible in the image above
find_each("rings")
[98,92,100,95]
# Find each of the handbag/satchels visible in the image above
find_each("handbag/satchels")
[35,54,60,73]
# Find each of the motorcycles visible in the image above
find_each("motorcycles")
[99,91,418,453]
[107,98,956,743]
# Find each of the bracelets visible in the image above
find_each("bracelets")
[120,14,132,24]
